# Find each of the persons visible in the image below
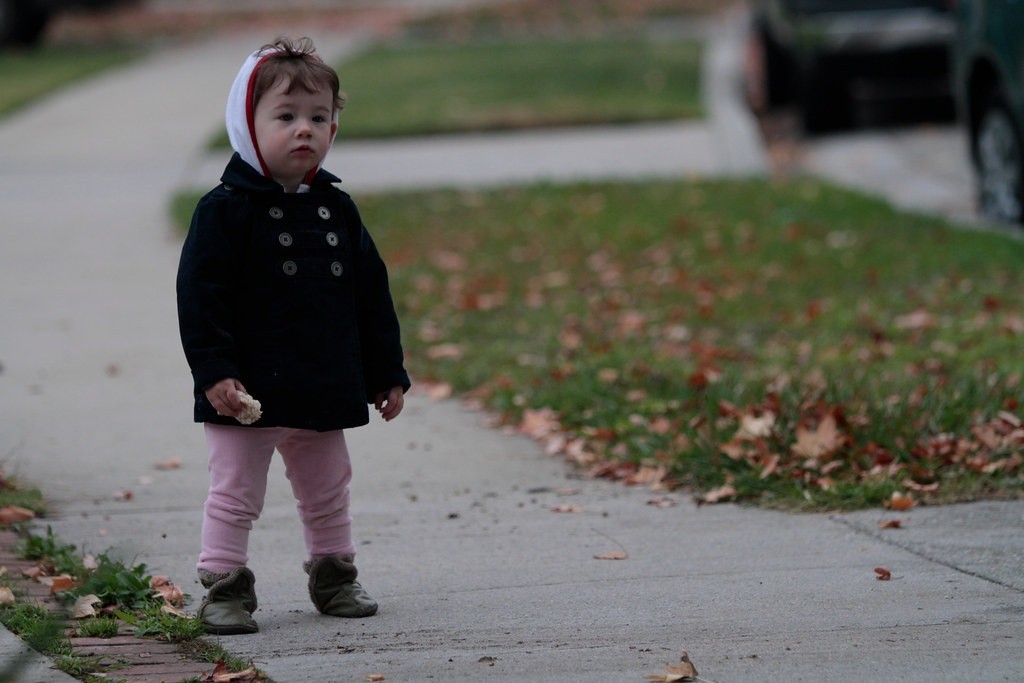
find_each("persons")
[176,35,411,631]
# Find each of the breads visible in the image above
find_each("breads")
[234,391,262,425]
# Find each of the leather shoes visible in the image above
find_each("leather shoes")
[196,569,258,635]
[303,562,377,617]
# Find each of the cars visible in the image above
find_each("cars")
[954,0,1023,231]
